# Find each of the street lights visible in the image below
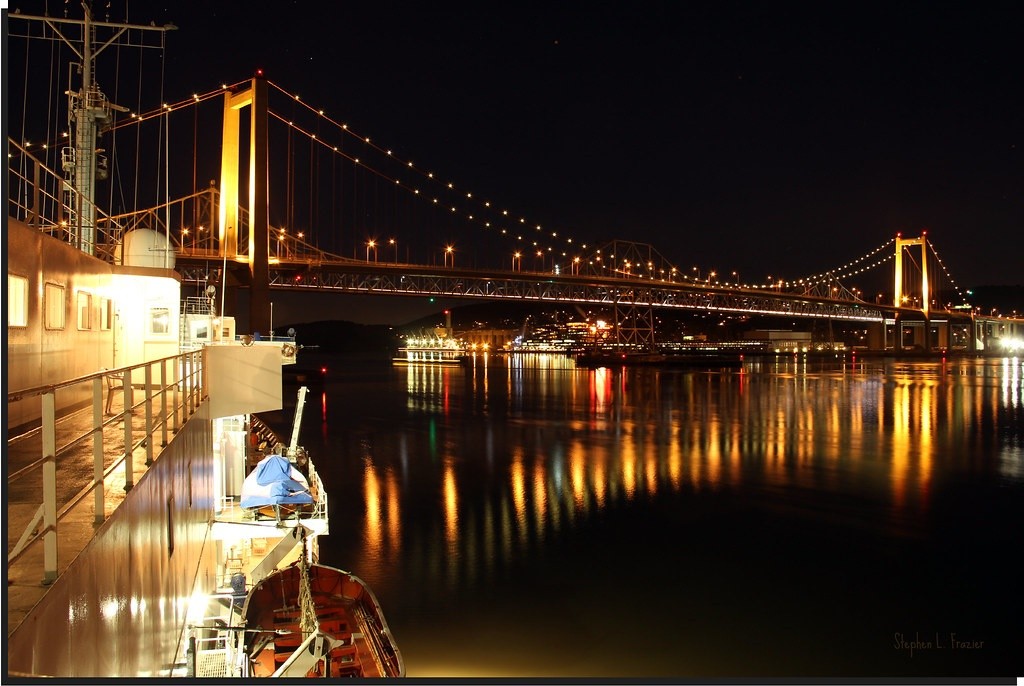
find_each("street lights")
[172,219,1023,321]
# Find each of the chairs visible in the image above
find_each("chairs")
[101,367,134,414]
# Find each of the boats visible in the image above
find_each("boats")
[2,1,289,680]
[240,520,407,678]
[568,347,748,368]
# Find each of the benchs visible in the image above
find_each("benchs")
[274,605,361,678]
